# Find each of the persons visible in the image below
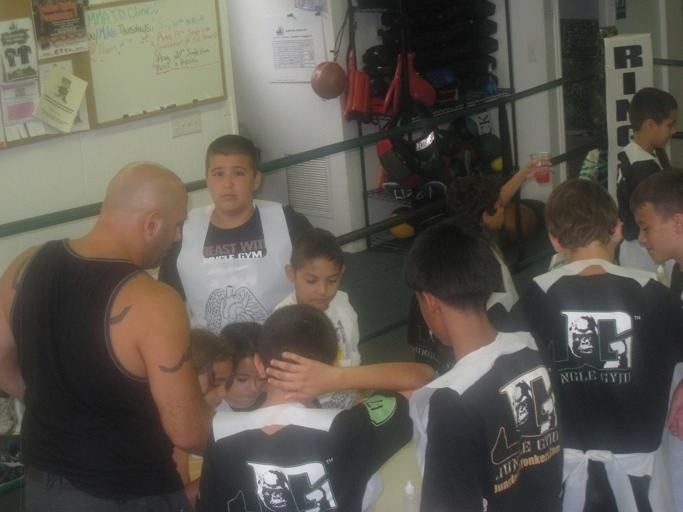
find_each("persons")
[160,133,436,512]
[445,156,537,278]
[616,87,678,242]
[408,218,564,512]
[0,158,217,510]
[524,168,682,512]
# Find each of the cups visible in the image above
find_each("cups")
[528,152,553,184]
